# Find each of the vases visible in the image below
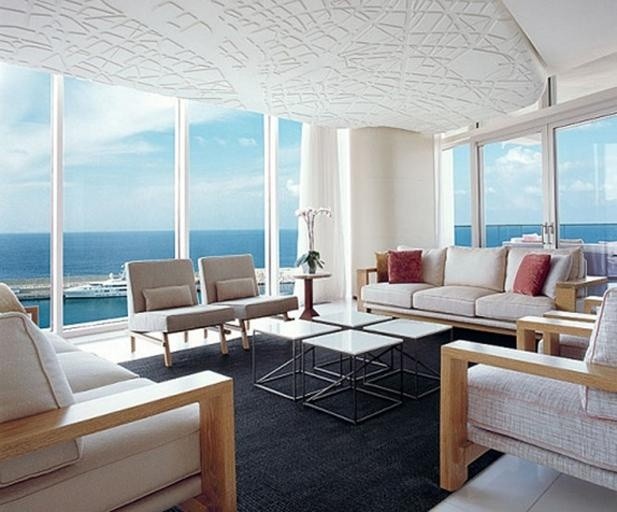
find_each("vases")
[307,261,316,274]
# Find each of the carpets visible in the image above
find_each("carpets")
[117,333,508,512]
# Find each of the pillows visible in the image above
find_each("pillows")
[142,284,194,311]
[579,282,617,423]
[216,277,256,301]
[0,282,28,314]
[375,245,582,300]
[0,311,83,490]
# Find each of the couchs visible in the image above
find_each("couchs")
[0,282,236,512]
[356,246,609,341]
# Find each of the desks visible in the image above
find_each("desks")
[511,237,583,244]
[581,242,617,277]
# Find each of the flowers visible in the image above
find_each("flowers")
[294,207,335,270]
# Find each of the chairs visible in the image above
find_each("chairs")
[125,259,235,368]
[537,295,603,361]
[197,254,299,350]
[439,287,617,492]
